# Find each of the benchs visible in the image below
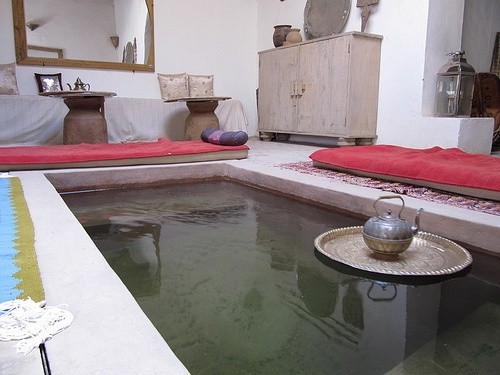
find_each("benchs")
[0,94,250,145]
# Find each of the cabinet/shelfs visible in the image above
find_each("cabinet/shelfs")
[257,32,384,147]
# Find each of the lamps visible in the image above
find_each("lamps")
[433,51,475,118]
[27,22,39,31]
[110,36,119,49]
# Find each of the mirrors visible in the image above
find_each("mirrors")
[12,0,155,73]
[27,45,64,59]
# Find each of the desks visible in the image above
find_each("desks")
[38,90,118,145]
[164,96,232,140]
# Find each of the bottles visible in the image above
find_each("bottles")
[273,25,292,48]
[286,28,302,43]
[184,101,219,141]
[62,97,109,144]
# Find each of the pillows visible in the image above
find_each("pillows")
[35,73,63,93]
[157,73,187,100]
[0,63,20,94]
[201,128,249,145]
[187,75,215,96]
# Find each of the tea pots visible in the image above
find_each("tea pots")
[66,77,91,91]
[361,195,424,258]
